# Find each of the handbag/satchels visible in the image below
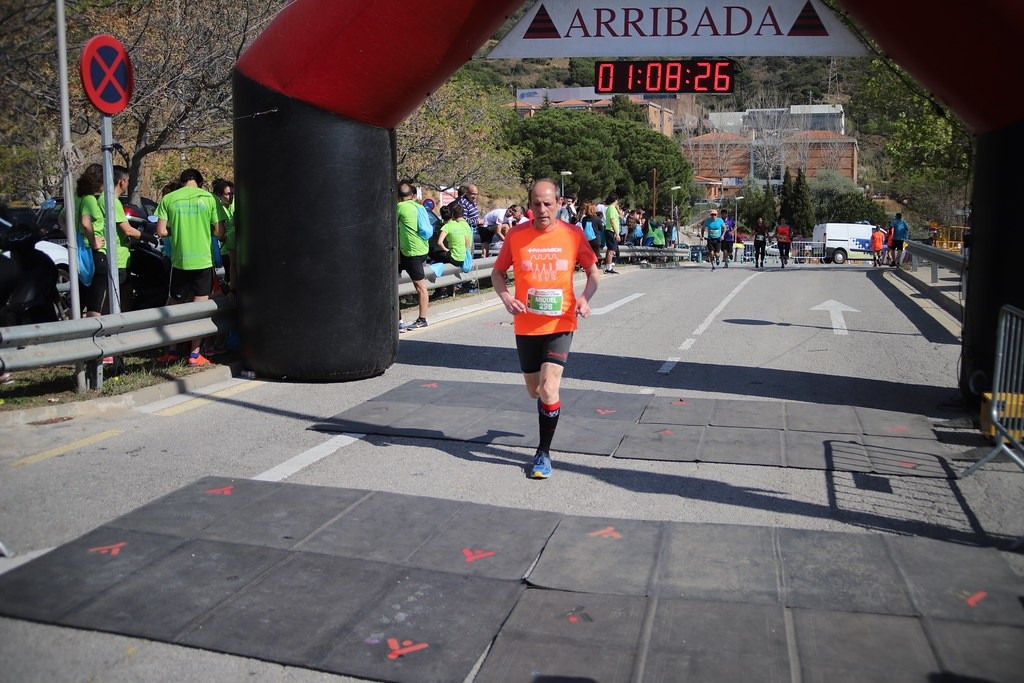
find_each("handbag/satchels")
[77,227,95,287]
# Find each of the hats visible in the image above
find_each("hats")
[895,213,902,217]
[710,209,718,215]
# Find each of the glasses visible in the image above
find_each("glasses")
[711,214,716,216]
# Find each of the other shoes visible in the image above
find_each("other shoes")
[716,258,720,266]
[711,267,716,272]
[755,263,758,268]
[723,264,728,268]
[874,257,899,267]
[785,260,787,265]
[429,275,511,301]
[729,254,733,260]
[760,262,763,267]
[782,264,784,268]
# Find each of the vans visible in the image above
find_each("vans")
[812,223,888,264]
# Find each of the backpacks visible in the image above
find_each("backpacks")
[636,224,644,237]
[463,249,472,272]
[400,201,434,239]
[584,221,596,240]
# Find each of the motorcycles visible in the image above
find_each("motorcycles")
[124,203,171,309]
[1,199,69,326]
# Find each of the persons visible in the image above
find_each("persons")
[557,193,621,274]
[162,182,222,355]
[478,205,529,283]
[752,218,772,268]
[58,163,123,364]
[448,184,487,259]
[153,168,216,365]
[871,225,885,267]
[429,204,473,301]
[398,183,429,332]
[491,178,601,478]
[700,209,727,272]
[771,216,794,268]
[524,203,535,220]
[97,165,158,317]
[886,213,908,267]
[721,210,737,269]
[211,175,234,282]
[616,204,676,263]
[220,210,237,296]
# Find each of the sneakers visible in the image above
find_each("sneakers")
[531,451,552,478]
[407,320,429,330]
[102,332,229,365]
[399,322,406,333]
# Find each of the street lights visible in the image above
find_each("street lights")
[670,186,682,222]
[734,196,744,242]
[560,171,572,197]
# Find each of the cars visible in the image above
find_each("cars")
[0,217,69,300]
[711,198,729,209]
[759,243,792,259]
[695,199,710,205]
